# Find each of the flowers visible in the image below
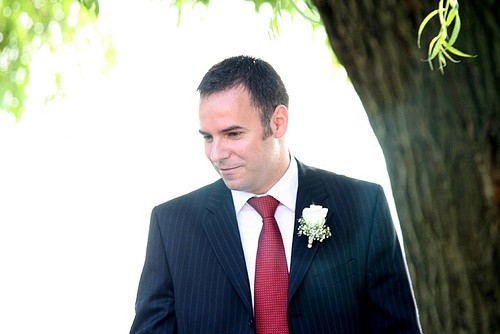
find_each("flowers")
[297,202,333,250]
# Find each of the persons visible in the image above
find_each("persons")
[129,55,422,334]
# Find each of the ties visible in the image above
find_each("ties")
[247,195,290,334]
